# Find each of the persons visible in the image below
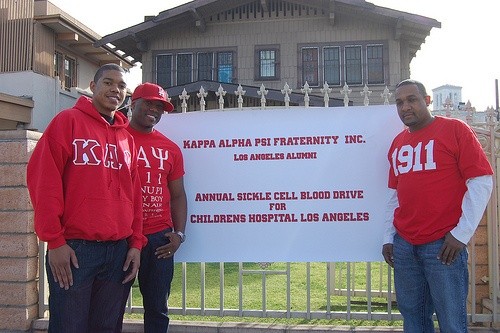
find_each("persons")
[380,78,495,333]
[27,63,148,333]
[118,82,188,332]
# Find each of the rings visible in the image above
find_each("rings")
[168,251,172,255]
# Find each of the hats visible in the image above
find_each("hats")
[131,82,174,112]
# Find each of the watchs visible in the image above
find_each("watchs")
[175,231,186,243]
